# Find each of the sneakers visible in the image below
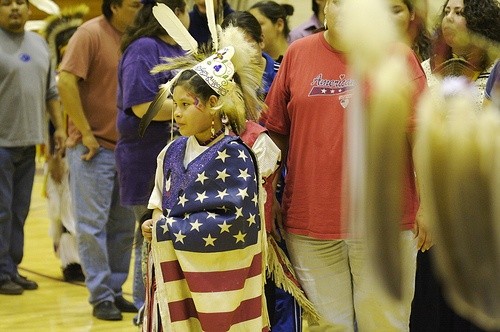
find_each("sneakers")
[64,262,87,282]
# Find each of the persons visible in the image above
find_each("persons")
[0,0,500,331]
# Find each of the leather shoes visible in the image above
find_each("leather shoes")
[92,295,138,321]
[0,273,38,295]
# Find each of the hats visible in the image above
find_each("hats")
[138,0,271,137]
[24,0,89,67]
